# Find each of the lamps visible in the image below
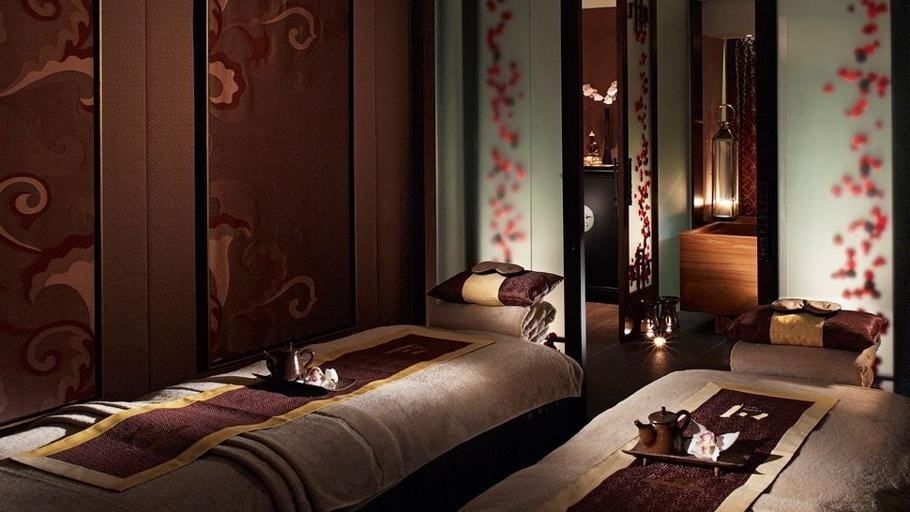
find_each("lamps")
[712,104,739,222]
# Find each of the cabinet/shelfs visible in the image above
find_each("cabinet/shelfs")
[583,165,618,306]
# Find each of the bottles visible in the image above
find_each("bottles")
[637,297,662,341]
[658,296,681,339]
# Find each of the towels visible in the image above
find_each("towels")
[729,338,881,389]
[431,301,555,345]
[210,324,584,511]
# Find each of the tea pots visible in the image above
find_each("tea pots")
[261,340,316,380]
[633,406,692,456]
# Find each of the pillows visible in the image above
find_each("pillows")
[729,297,883,352]
[427,260,566,308]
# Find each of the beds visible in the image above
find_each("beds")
[460,370,910,512]
[0,325,585,512]
